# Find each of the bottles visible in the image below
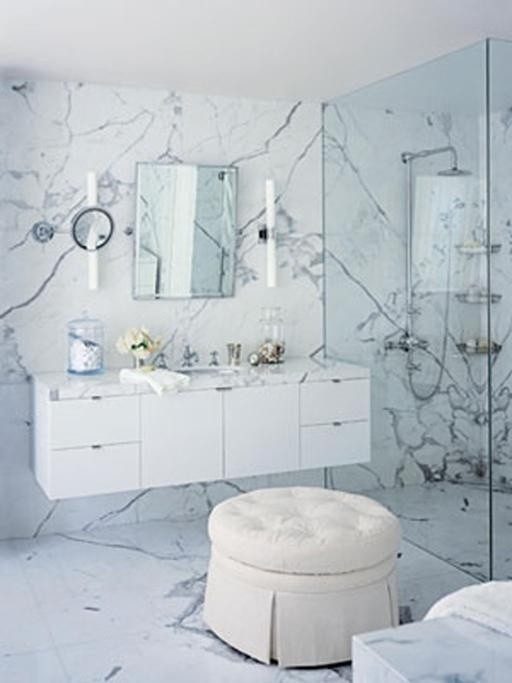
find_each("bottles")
[257,301,285,361]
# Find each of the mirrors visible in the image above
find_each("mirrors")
[134,161,238,300]
[31,206,114,250]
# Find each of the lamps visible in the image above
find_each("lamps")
[257,179,277,289]
[86,171,100,291]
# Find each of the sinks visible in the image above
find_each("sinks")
[173,367,258,384]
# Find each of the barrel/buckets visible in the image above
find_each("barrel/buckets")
[66,318,105,376]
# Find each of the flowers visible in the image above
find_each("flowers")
[115,327,160,357]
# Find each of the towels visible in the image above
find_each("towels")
[119,366,191,395]
[423,579,512,636]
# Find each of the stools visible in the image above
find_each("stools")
[201,485,403,669]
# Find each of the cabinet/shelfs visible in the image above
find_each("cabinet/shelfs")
[30,356,372,502]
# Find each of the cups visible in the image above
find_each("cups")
[67,309,105,372]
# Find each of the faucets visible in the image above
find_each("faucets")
[181,345,198,368]
[209,349,218,367]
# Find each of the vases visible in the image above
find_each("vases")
[132,357,145,367]
